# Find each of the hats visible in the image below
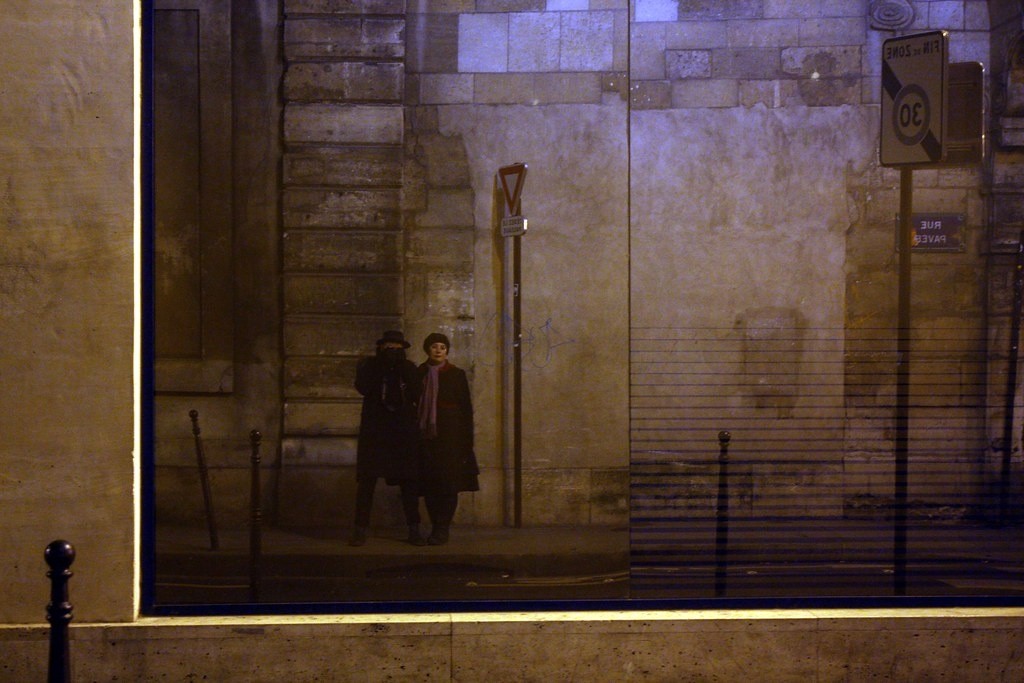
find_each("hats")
[375,330,411,349]
[424,332,449,353]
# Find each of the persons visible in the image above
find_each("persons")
[417,333,479,546]
[349,330,426,547]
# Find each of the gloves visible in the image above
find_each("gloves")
[381,346,406,368]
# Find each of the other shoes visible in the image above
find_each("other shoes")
[408,532,425,545]
[351,531,365,545]
[427,523,448,543]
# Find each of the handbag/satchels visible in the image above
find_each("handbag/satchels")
[463,446,480,474]
